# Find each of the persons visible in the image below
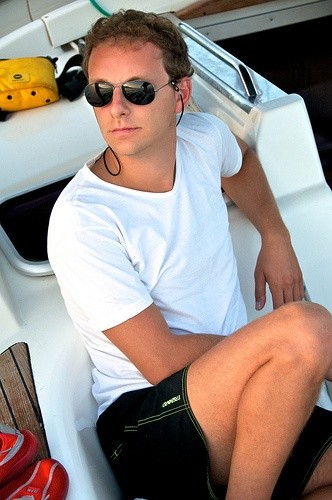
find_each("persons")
[47,8,332,500]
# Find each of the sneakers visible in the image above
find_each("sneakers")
[0,423,39,488]
[0,459,69,500]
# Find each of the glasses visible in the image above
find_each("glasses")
[83,80,172,107]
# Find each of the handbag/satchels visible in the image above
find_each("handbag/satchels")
[0,54,88,122]
